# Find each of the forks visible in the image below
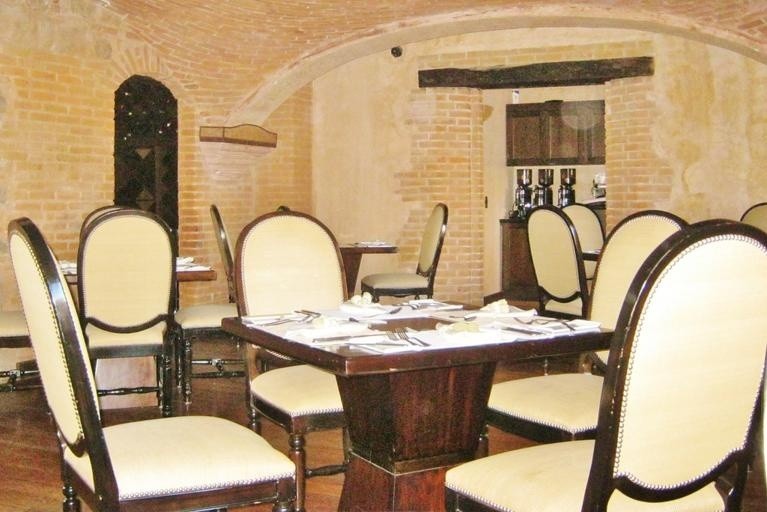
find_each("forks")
[514,316,576,326]
[385,327,433,348]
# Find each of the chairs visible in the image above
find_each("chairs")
[230,211,357,512]
[523,206,591,320]
[70,211,180,418]
[439,218,767,511]
[483,206,692,443]
[0,203,767,512]
[360,204,450,303]
[4,212,301,512]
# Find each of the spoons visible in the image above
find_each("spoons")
[447,312,478,322]
[373,306,403,314]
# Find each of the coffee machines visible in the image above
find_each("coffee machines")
[515,168,576,220]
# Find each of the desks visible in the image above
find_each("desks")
[217,300,619,510]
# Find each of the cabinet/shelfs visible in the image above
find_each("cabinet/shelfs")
[505,100,605,165]
